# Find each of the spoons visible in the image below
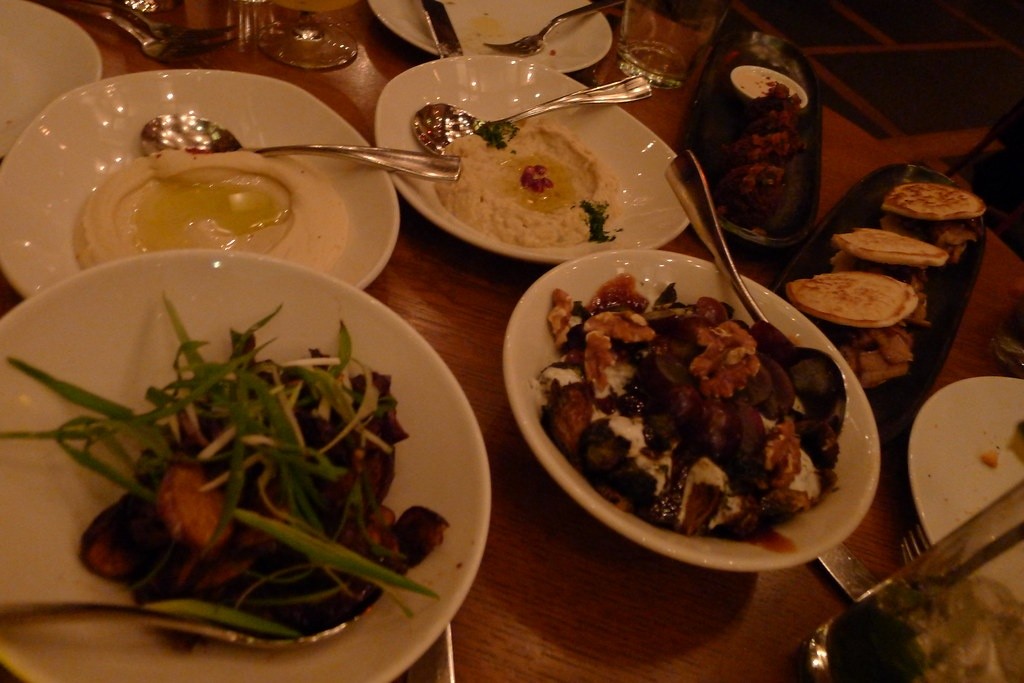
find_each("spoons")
[664,150,848,451]
[412,75,651,156]
[137,115,463,181]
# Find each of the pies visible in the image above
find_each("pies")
[784,183,986,385]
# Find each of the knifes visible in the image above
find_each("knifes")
[815,542,882,602]
[420,0,463,58]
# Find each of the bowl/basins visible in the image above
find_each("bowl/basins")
[728,64,809,111]
[504,248,881,571]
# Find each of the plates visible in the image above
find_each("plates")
[770,162,985,447]
[0,0,102,161]
[0,249,491,683]
[373,56,691,262]
[688,30,821,246]
[908,376,1024,544]
[2,70,400,298]
[367,0,612,72]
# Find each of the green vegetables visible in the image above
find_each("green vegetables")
[571,199,623,242]
[474,119,520,149]
[0,291,439,638]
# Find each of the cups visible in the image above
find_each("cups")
[617,1,729,87]
[798,472,1024,683]
[155,1,240,50]
[250,1,365,69]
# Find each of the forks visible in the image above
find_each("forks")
[33,0,239,59]
[1,593,371,649]
[483,0,625,58]
[899,523,930,567]
